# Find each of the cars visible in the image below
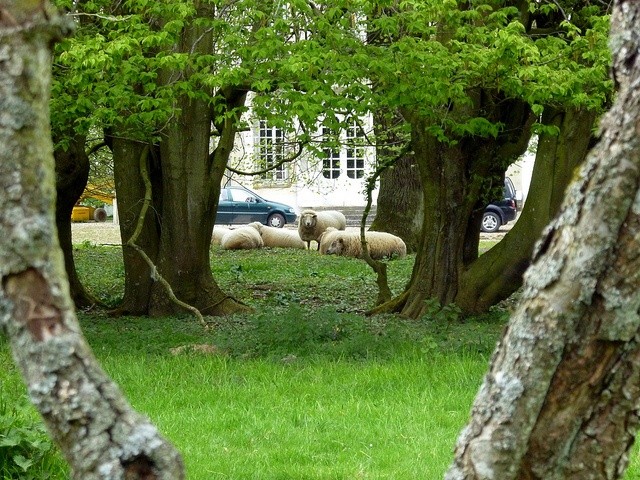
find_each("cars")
[214,186,297,228]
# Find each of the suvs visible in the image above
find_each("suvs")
[480,176,523,232]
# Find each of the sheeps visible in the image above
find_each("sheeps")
[213,224,241,242]
[319,226,368,256]
[253,221,307,250]
[220,224,266,250]
[326,231,406,260]
[298,209,346,251]
